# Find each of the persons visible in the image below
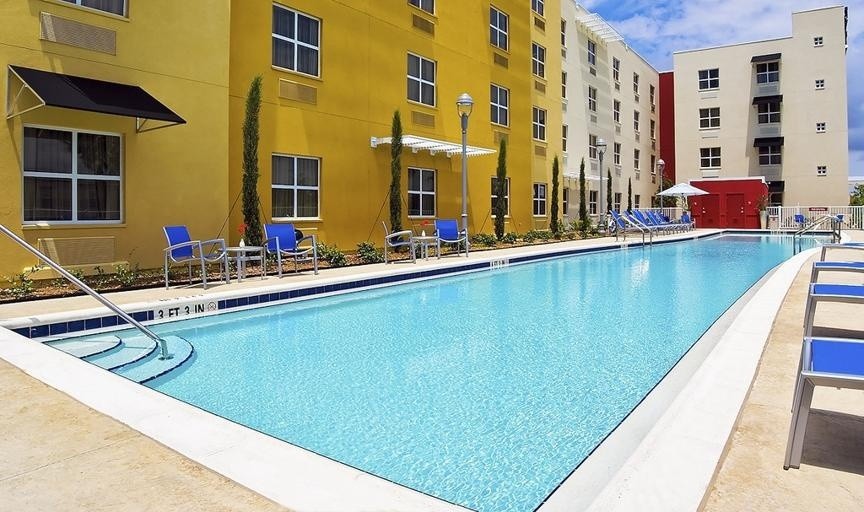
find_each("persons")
[680,210,695,231]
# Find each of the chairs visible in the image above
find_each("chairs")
[832,215,844,229]
[160,224,318,290]
[794,215,807,233]
[382,218,468,264]
[609,210,695,241]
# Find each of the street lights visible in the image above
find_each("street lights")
[594,139,607,234]
[455,91,474,249]
[657,158,665,217]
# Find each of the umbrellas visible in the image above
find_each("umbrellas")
[656,182,711,211]
